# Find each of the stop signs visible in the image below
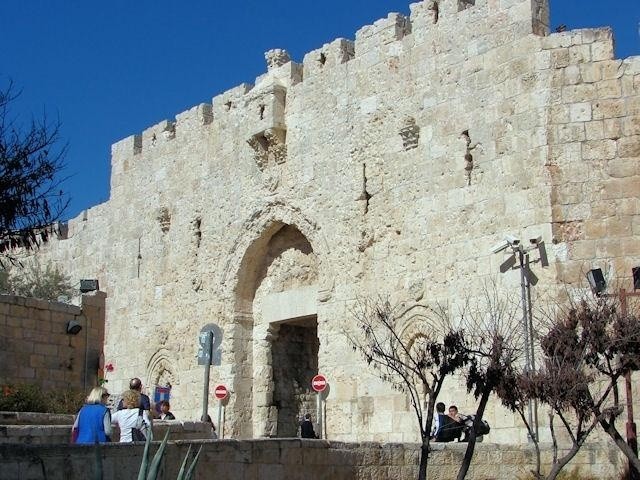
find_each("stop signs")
[214,385,228,400]
[312,375,327,391]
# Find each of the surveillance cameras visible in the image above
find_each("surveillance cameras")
[504,232,520,248]
[488,240,509,255]
[528,234,543,246]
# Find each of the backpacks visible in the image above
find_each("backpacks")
[440,415,490,438]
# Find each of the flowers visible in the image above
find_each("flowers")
[96,363,113,386]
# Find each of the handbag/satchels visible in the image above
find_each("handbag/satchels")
[132,428,146,441]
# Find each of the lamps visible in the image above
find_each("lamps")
[80,279,100,293]
[67,320,82,334]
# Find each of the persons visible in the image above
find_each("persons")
[72,378,175,444]
[430,402,490,442]
[201,414,216,431]
[301,414,315,439]
